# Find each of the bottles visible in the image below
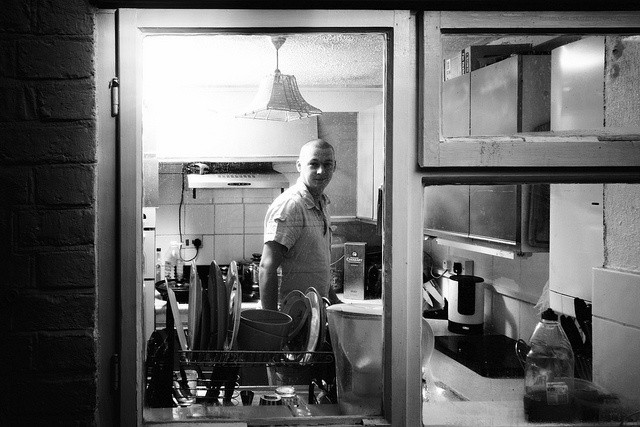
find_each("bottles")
[524,307,576,423]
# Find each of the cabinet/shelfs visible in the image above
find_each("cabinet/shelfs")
[177,327,335,366]
[423,54,551,257]
[356,105,385,224]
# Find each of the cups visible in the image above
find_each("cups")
[275,385,297,406]
[172,370,198,405]
[259,394,283,407]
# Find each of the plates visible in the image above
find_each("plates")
[164,277,189,364]
[208,261,227,361]
[300,287,325,366]
[224,260,242,351]
[188,259,203,349]
[281,290,312,362]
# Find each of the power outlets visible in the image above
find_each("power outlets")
[444,259,467,275]
[184,237,203,249]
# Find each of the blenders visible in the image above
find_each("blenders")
[325,299,434,414]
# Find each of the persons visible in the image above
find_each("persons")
[259,140,344,384]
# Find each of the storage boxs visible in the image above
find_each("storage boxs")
[441,44,532,82]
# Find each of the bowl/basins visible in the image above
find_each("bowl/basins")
[238,309,293,364]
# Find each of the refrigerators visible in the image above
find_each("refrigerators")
[142,207,156,363]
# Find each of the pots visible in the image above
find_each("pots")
[154,280,190,297]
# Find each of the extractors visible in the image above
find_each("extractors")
[186,162,289,189]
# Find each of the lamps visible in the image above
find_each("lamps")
[234,39,324,122]
